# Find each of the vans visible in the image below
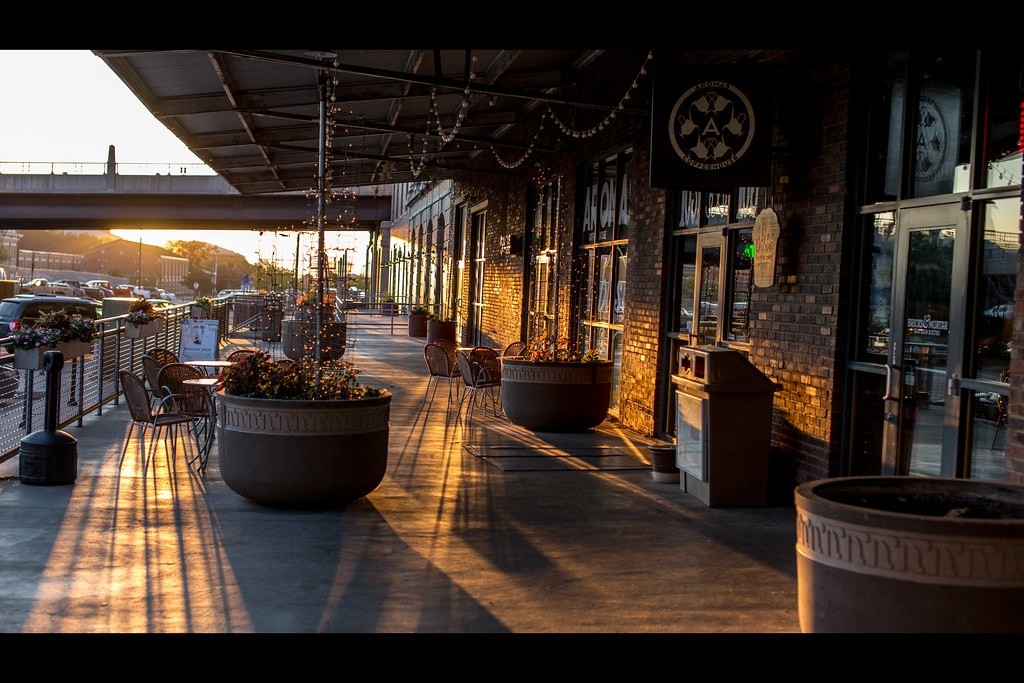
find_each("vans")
[218,289,256,301]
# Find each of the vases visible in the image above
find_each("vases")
[217,386,392,510]
[13,337,93,370]
[792,474,1023,635]
[280,320,347,361]
[500,357,613,430]
[124,318,161,338]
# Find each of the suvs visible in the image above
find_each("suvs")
[0,294,104,354]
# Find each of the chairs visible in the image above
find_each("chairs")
[144,346,218,475]
[419,338,529,423]
[117,369,204,478]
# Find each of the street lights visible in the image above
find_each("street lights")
[254,251,261,293]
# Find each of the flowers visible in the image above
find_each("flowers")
[217,347,385,399]
[6,307,102,350]
[517,336,603,365]
[123,296,165,326]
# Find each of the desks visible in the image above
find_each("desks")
[454,347,502,356]
[184,360,238,378]
[182,377,221,475]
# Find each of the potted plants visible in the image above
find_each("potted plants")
[191,296,213,318]
[381,292,400,316]
[426,316,457,344]
[408,305,432,337]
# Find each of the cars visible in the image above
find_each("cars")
[0,268,175,308]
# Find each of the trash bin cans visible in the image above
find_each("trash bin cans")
[261,298,282,343]
[670,344,784,508]
